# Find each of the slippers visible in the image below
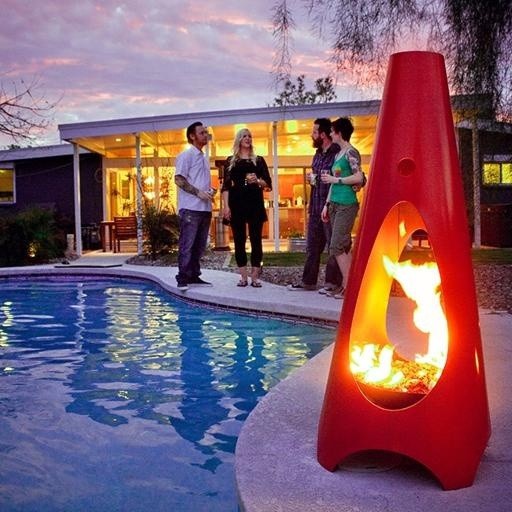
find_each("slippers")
[237,279,262,287]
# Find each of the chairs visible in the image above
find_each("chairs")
[112,216,137,253]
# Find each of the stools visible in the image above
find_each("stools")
[100,221,115,251]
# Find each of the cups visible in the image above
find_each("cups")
[245,172,257,184]
[320,169,330,181]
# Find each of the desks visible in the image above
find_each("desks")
[262,207,305,238]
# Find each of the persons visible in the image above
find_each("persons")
[224,326,268,429]
[286,116,367,295]
[220,128,273,288]
[166,307,230,476]
[174,120,219,291]
[319,115,363,299]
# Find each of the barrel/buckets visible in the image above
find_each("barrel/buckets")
[67,233,75,249]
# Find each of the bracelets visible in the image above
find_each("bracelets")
[338,176,342,184]
[325,201,331,207]
[256,178,260,184]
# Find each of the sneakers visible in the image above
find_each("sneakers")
[177,276,213,291]
[287,284,314,291]
[318,286,345,299]
